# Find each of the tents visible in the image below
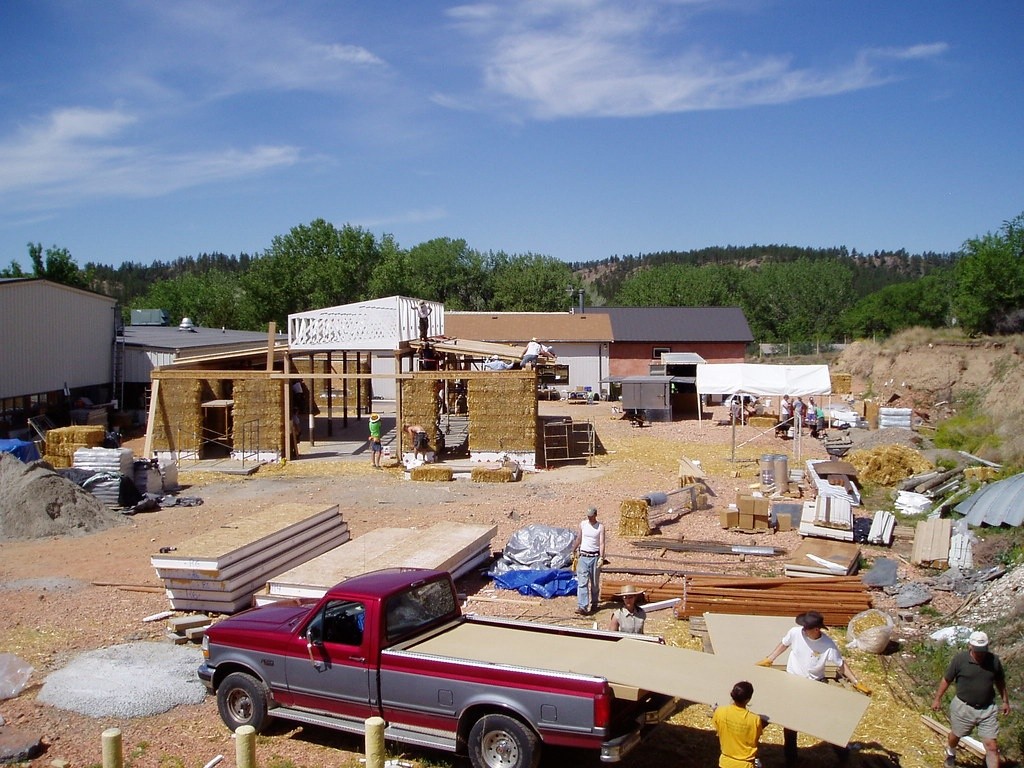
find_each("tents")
[696,363,832,429]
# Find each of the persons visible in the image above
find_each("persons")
[404,424,428,461]
[572,507,605,614]
[416,342,437,371]
[369,414,381,468]
[485,355,515,371]
[815,404,825,438]
[519,337,552,367]
[711,680,763,768]
[931,631,1011,768]
[610,585,665,645]
[411,300,432,340]
[808,397,816,433]
[793,397,803,433]
[781,395,790,434]
[758,611,873,768]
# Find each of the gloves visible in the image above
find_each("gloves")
[853,681,872,694]
[755,658,772,667]
[571,553,576,560]
[596,556,603,569]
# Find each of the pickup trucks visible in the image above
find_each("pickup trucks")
[195,565,680,768]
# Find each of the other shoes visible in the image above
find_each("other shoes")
[943,748,956,768]
[591,603,598,611]
[575,607,585,615]
[784,752,798,768]
[837,750,849,764]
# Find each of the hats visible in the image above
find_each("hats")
[532,337,539,342]
[614,585,643,596]
[369,414,380,422]
[586,506,597,516]
[419,300,425,306]
[795,610,829,630]
[784,395,790,399]
[490,355,498,360]
[968,631,989,647]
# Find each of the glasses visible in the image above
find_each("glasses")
[802,626,813,631]
[622,595,635,599]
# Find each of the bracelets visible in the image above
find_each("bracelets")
[1003,701,1008,703]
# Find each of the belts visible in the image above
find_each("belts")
[959,698,992,710]
